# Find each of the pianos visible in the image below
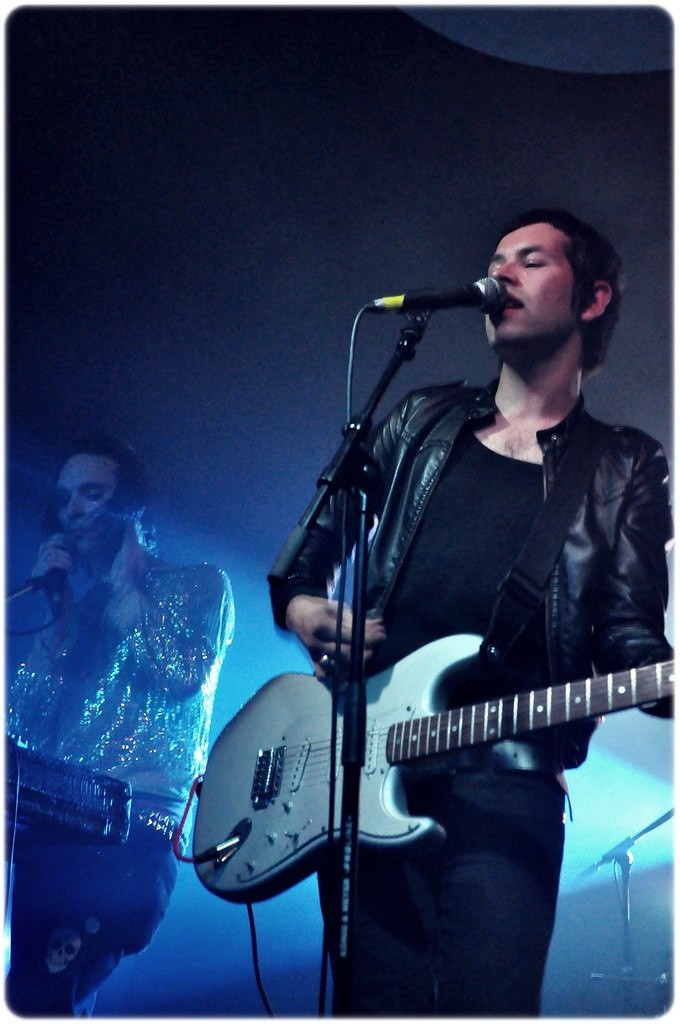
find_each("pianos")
[6,731,132,848]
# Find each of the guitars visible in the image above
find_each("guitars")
[192,632,674,904]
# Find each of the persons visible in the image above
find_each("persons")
[266,205,675,1019]
[6,432,237,1017]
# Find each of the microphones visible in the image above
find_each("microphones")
[363,276,507,314]
[47,533,75,622]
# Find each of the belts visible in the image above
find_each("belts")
[131,806,191,857]
[399,740,566,777]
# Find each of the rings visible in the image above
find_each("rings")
[319,654,330,668]
